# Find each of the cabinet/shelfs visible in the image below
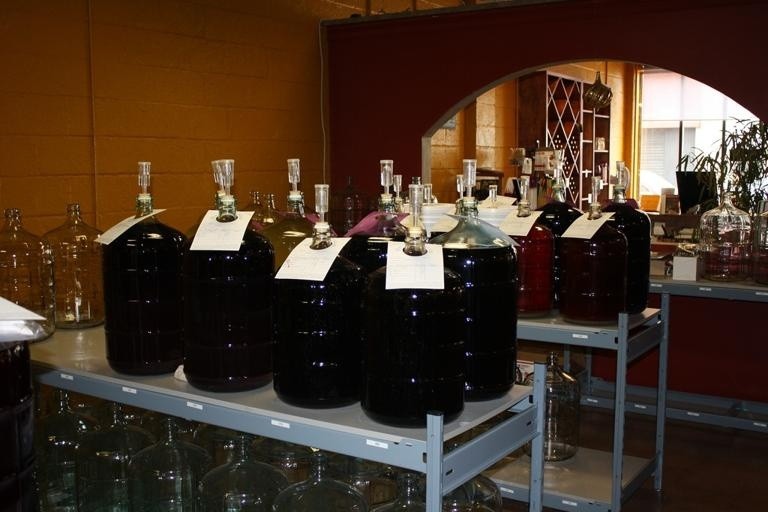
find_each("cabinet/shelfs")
[516,69,612,214]
[562,272,768,431]
[479,284,671,511]
[30,318,547,511]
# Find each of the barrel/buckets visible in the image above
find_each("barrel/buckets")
[182,159,267,393]
[106,163,177,374]
[753,201,768,284]
[564,181,625,322]
[42,202,104,332]
[249,157,436,262]
[477,184,509,220]
[273,183,359,411]
[509,172,551,354]
[1,207,56,345]
[439,157,522,398]
[364,184,459,420]
[609,161,651,311]
[699,192,753,283]
